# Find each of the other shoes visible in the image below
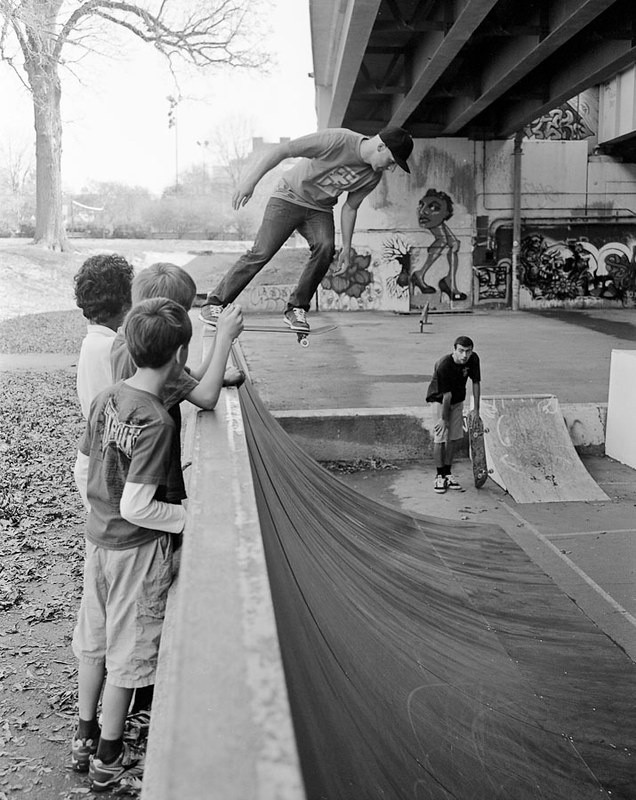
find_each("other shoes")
[123,709,151,742]
[433,474,447,493]
[445,474,461,489]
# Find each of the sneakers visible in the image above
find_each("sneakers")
[89,743,146,791]
[70,730,100,772]
[284,308,310,331]
[198,305,224,325]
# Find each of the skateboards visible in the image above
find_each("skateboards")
[199,313,339,347]
[467,410,494,488]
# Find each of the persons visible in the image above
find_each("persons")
[429,335,482,494]
[71,298,191,793]
[72,253,242,582]
[200,128,415,331]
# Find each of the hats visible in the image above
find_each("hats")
[377,126,414,174]
